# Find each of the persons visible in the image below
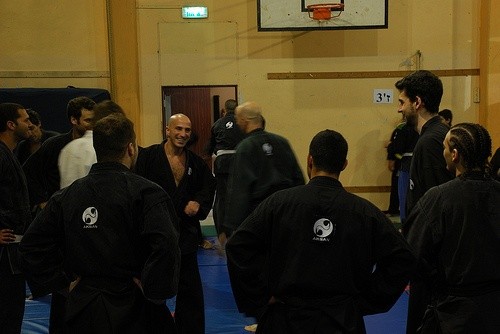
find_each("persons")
[20,112,181,334]
[57,101,126,190]
[403,123,500,334]
[17,109,61,164]
[394,69,456,224]
[219,99,307,332]
[22,97,98,208]
[0,102,34,334]
[382,117,412,220]
[133,114,217,334]
[438,108,453,129]
[206,99,239,246]
[225,129,410,334]
